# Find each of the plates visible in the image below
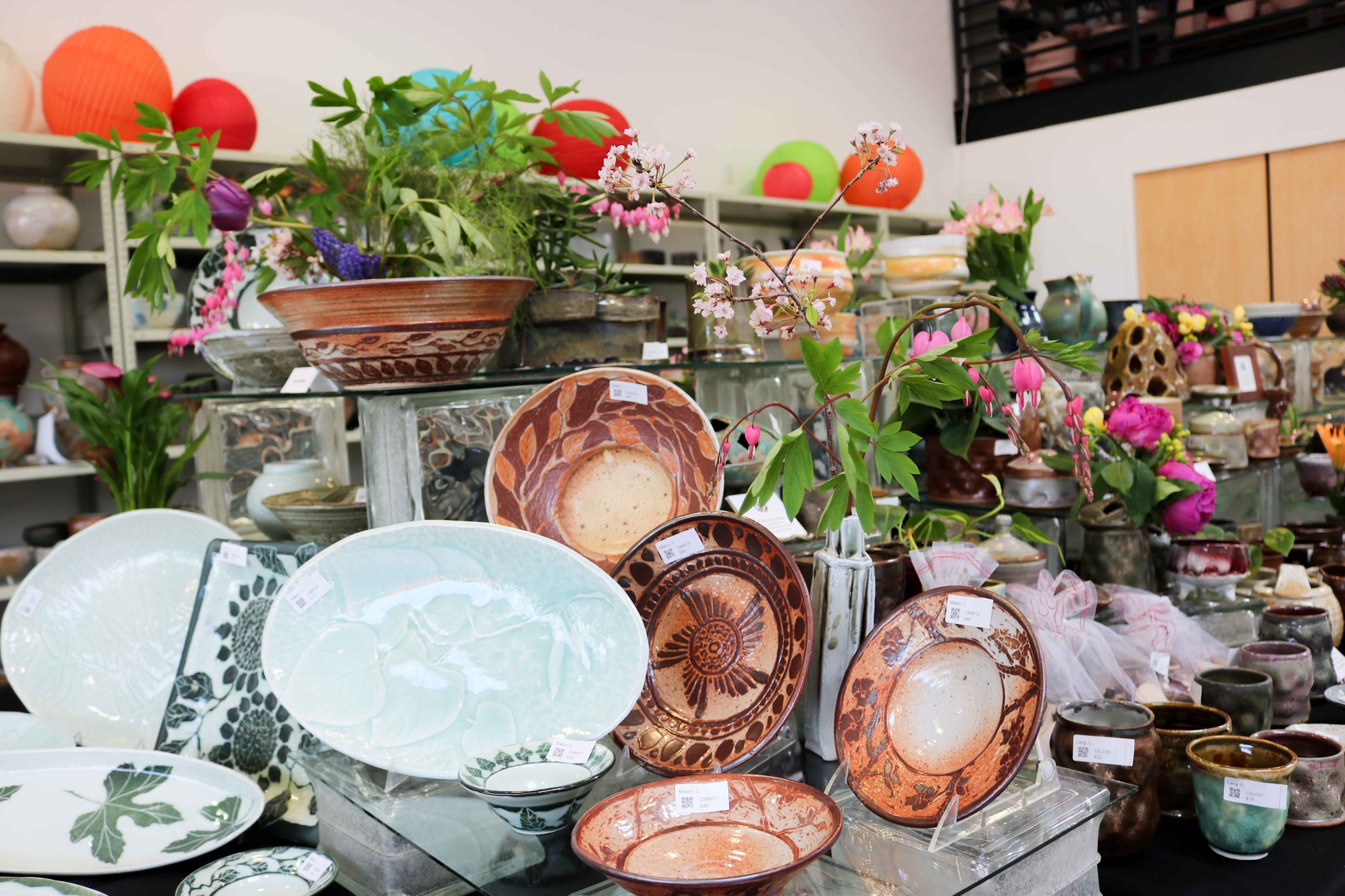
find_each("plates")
[1324,684,1345,705]
[608,511,814,773]
[0,508,339,896]
[834,586,1045,828]
[187,228,341,380]
[261,520,650,780]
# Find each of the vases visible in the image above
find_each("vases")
[1326,298,1345,336]
[527,289,662,368]
[2,185,82,249]
[802,508,876,761]
[997,288,1046,356]
[1178,351,1217,387]
[252,276,547,385]
[924,416,1006,503]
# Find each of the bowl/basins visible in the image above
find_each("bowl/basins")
[1103,300,1174,344]
[1242,305,1331,342]
[458,740,615,835]
[0,515,103,577]
[571,773,844,896]
[204,328,311,388]
[256,277,537,390]
[262,484,368,542]
[739,235,969,315]
[484,366,726,574]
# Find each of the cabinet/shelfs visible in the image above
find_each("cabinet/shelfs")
[109,140,942,518]
[0,130,124,598]
[167,357,1345,896]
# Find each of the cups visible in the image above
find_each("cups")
[1142,605,1345,861]
[1320,563,1345,610]
[1245,419,1280,458]
[1139,397,1183,443]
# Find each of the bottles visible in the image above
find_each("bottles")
[247,458,341,541]
[1050,700,1162,857]
[0,323,108,468]
[4,188,80,250]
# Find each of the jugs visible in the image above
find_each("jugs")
[1041,275,1108,349]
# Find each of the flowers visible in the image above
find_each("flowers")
[1318,259,1345,299]
[62,66,685,357]
[27,353,231,513]
[598,118,1251,538]
